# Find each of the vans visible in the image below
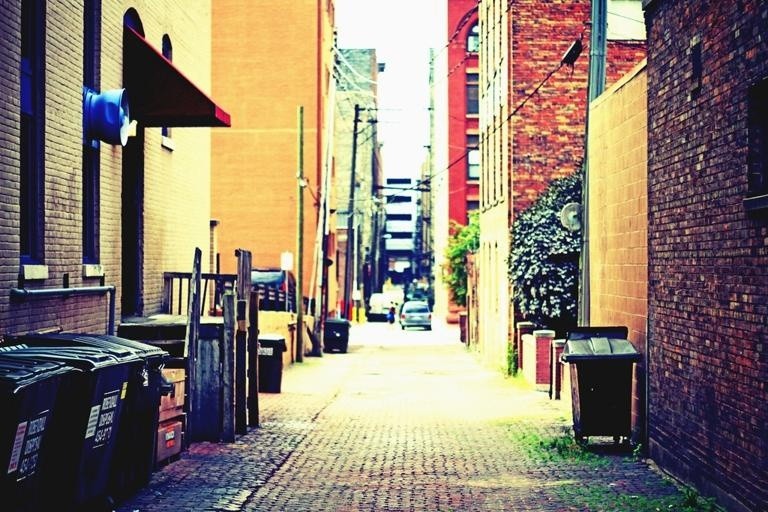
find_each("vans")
[366,292,396,323]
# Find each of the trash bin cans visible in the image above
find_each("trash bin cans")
[324,320,349,354]
[559,326,643,445]
[0,331,170,511]
[258,335,286,394]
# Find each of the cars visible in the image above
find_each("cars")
[399,301,431,331]
[232,267,298,312]
